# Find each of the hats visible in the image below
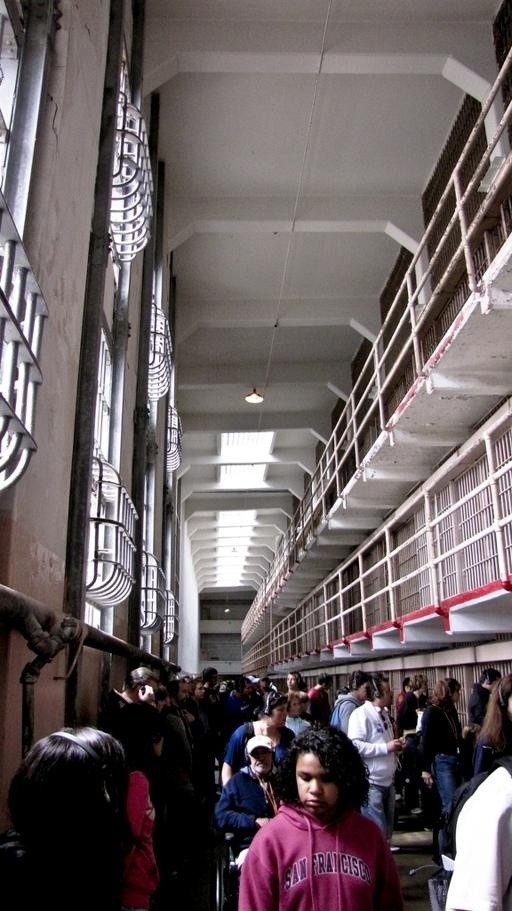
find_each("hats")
[248,676,261,684]
[246,736,275,753]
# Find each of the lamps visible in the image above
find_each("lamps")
[245,387,264,404]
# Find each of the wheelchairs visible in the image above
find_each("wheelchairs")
[216,791,240,911]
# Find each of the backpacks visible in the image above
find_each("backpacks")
[428,755,512,911]
[432,754,512,885]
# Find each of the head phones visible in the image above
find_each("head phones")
[244,736,276,766]
[352,671,360,689]
[136,667,146,696]
[441,678,453,699]
[264,691,273,714]
[485,668,492,685]
[51,730,129,818]
[498,679,507,712]
[372,677,382,698]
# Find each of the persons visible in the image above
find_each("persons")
[186,678,216,812]
[467,668,502,752]
[284,672,308,696]
[421,678,462,867]
[334,686,350,699]
[214,735,281,888]
[220,693,296,788]
[398,674,429,814]
[0,727,132,911]
[202,667,226,719]
[121,729,160,911]
[281,692,312,736]
[393,677,409,801]
[306,671,333,726]
[105,667,169,767]
[512,674,512,911]
[331,670,370,739]
[298,691,308,716]
[229,688,239,696]
[155,684,166,714]
[346,674,408,851]
[168,679,195,765]
[258,677,278,713]
[238,728,406,911]
[179,675,194,695]
[224,677,257,726]
